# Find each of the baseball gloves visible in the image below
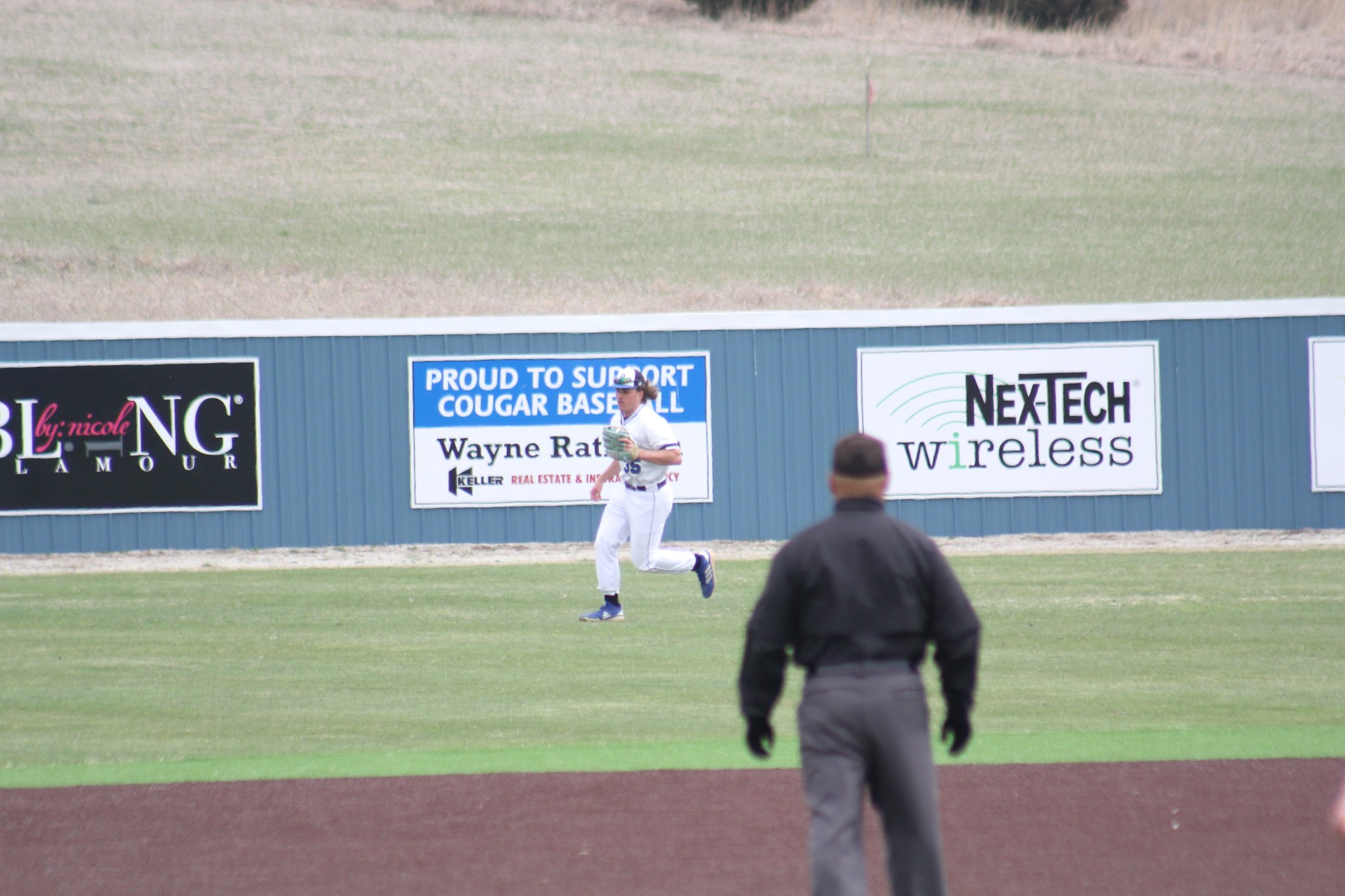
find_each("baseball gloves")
[602,425,640,463]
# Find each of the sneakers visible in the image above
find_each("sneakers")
[692,550,716,599]
[579,601,625,622]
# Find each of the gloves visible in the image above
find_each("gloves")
[940,705,970,754]
[745,717,775,757]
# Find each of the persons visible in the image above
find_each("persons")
[579,368,715,620]
[740,434,983,896]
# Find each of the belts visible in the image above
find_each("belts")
[624,480,667,491]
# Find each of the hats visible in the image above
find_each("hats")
[834,434,886,478]
[614,367,645,388]
